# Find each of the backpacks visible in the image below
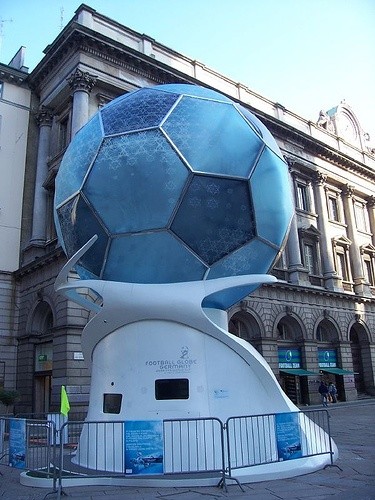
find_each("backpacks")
[331,385,337,394]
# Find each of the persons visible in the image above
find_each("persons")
[318,381,329,407]
[329,382,338,404]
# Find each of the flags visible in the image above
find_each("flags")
[60,386,70,415]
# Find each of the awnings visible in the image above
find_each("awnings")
[318,367,354,376]
[279,368,324,377]
